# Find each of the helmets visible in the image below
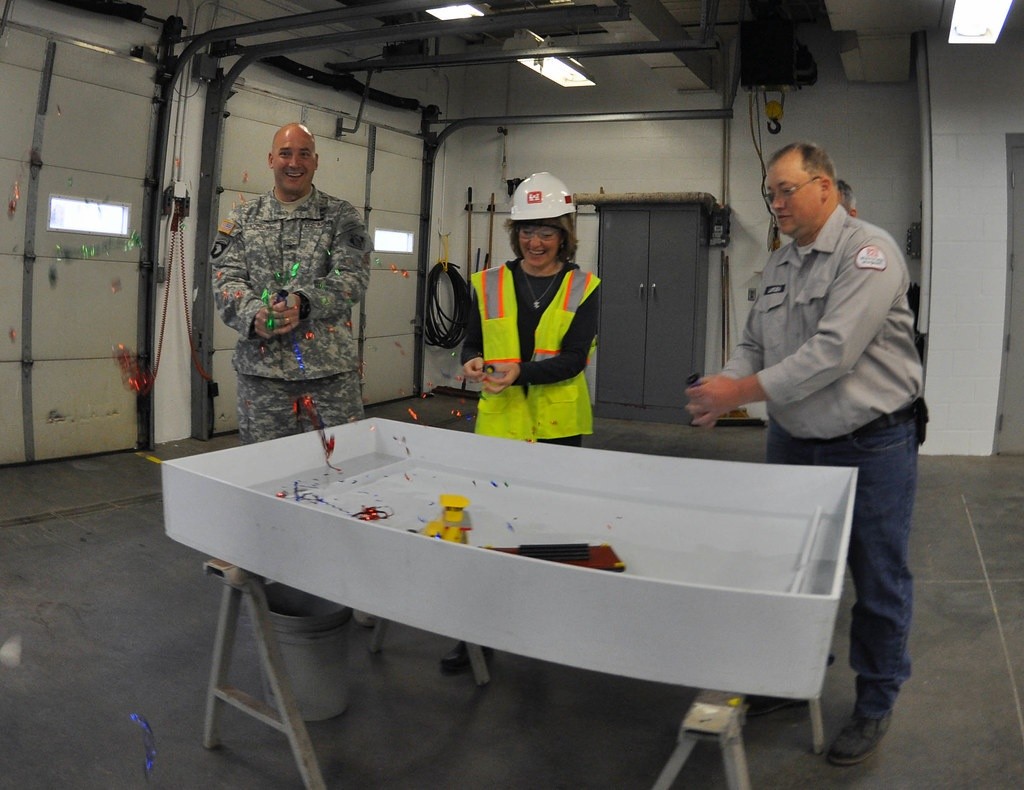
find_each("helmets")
[510,172,576,220]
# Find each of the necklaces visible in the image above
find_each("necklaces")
[522,261,561,308]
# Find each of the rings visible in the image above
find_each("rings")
[285,317,289,324]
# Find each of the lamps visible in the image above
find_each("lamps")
[423,0,597,87]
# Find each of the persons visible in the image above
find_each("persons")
[438,173,601,677]
[685,141,923,766]
[211,123,377,624]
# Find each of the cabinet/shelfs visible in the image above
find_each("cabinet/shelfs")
[593,197,710,425]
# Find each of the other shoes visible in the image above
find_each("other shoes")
[824,702,896,766]
[440,640,494,669]
[744,694,809,717]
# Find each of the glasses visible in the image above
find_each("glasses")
[764,176,822,203]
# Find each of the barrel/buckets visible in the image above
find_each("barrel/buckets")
[261,580,354,721]
